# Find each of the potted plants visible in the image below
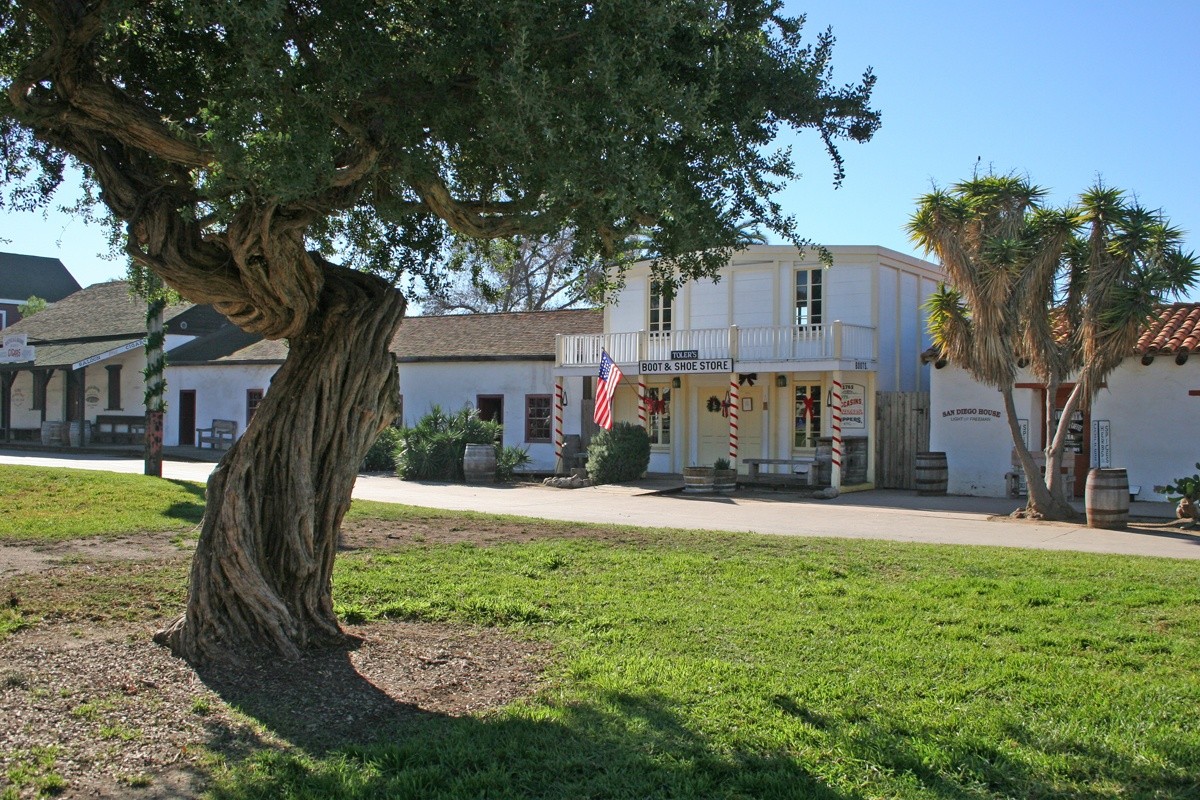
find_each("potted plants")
[714,458,737,492]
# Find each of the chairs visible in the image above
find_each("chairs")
[196,419,236,450]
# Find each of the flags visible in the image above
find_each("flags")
[594,352,622,432]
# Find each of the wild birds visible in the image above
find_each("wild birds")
[543,474,578,487]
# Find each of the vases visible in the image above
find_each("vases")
[681,466,714,492]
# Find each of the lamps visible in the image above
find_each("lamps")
[778,376,786,387]
[672,377,680,388]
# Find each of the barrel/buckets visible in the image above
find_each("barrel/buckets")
[40,421,61,447]
[714,469,738,494]
[463,443,496,485]
[69,421,91,448]
[683,467,714,493]
[562,434,581,473]
[1085,467,1130,530]
[915,451,948,495]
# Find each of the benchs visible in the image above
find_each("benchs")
[573,452,588,468]
[95,414,147,445]
[743,459,824,486]
[1007,449,1075,503]
